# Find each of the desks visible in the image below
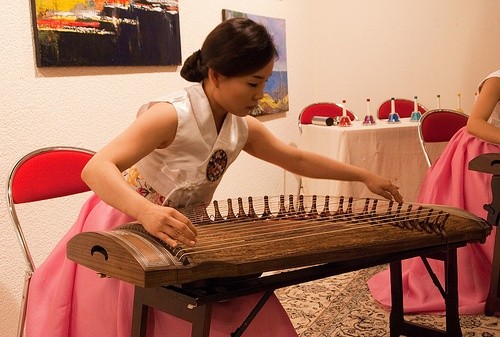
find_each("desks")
[278,118,451,203]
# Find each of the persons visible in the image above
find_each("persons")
[25,17,403,337]
[366,69,500,314]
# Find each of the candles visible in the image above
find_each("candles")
[391,98,394,113]
[367,99,370,115]
[343,100,346,116]
[437,95,440,109]
[475,93,477,102]
[457,94,460,109]
[414,96,418,111]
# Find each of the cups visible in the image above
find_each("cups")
[311,116,333,126]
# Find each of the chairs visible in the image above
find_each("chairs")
[378,98,428,120]
[298,102,358,124]
[418,109,469,167]
[6,146,97,337]
